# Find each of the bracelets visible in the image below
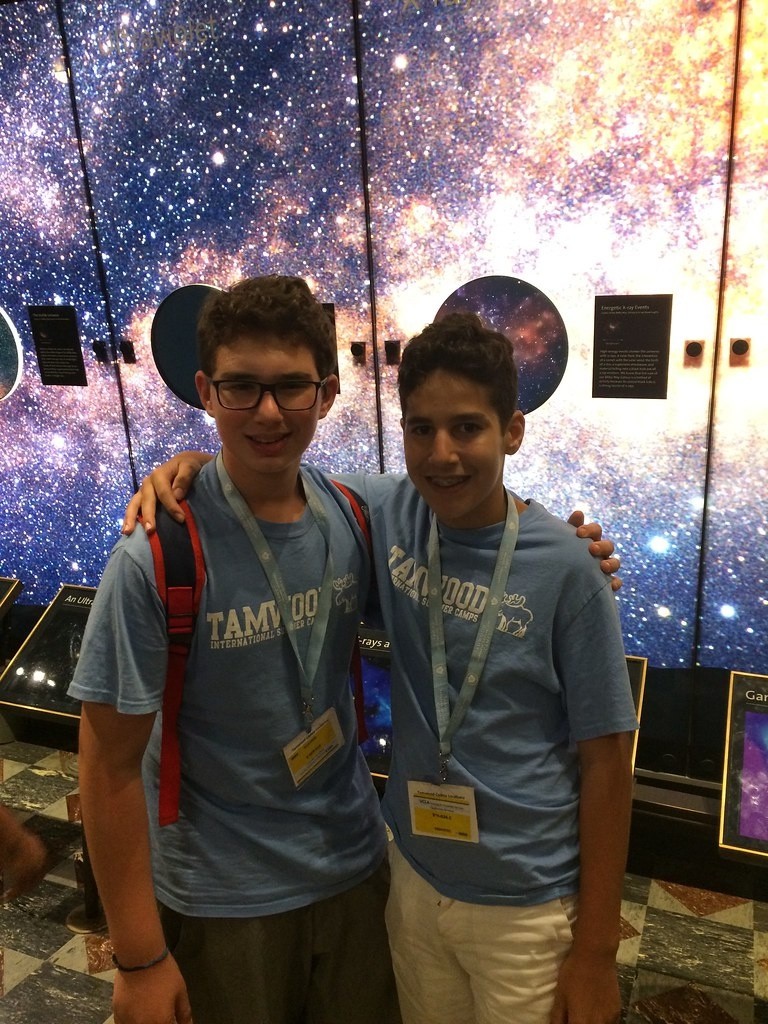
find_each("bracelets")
[112,947,169,973]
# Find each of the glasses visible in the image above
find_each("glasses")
[208,376,328,411]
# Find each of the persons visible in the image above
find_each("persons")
[122,310,641,1024]
[66,277,623,1024]
